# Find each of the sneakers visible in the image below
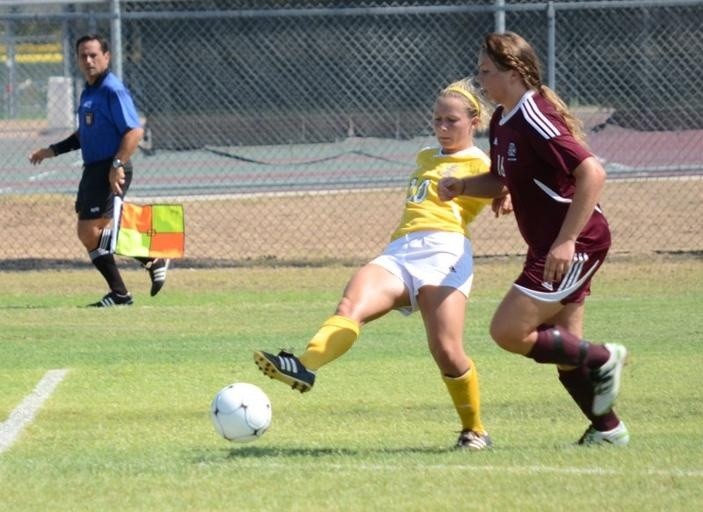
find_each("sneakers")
[141,259,170,297]
[253,350,316,393]
[590,342,627,416]
[454,430,492,454]
[555,422,629,454]
[86,290,134,308]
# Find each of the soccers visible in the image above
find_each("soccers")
[211,382,272,443]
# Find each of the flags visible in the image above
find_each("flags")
[110,194,185,260]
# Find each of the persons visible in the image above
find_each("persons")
[252,80,513,451]
[435,31,629,445]
[28,35,171,308]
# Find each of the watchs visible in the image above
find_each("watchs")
[112,158,126,169]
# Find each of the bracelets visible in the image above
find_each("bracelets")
[460,177,466,196]
[50,134,81,156]
